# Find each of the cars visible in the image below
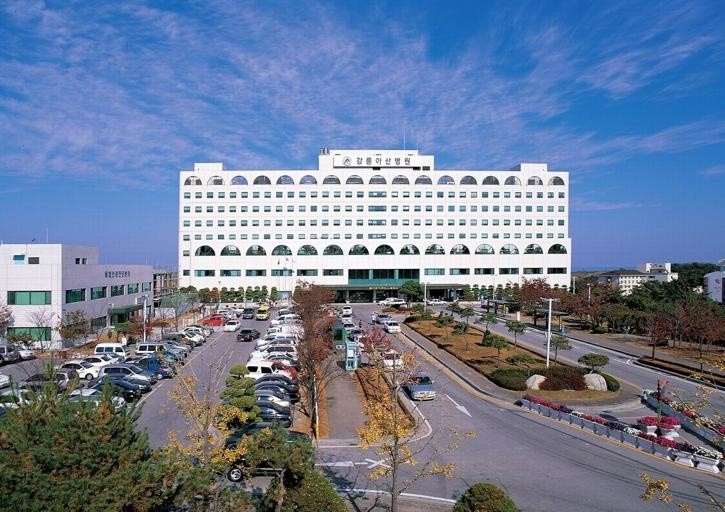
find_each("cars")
[384,320,401,334]
[376,314,391,324]
[402,371,437,401]
[340,314,374,353]
[380,347,404,373]
[341,306,353,316]
[378,297,407,308]
[427,298,448,306]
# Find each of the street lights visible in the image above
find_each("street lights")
[541,297,561,369]
[585,282,594,320]
[419,280,430,313]
[142,293,151,342]
[217,280,222,303]
